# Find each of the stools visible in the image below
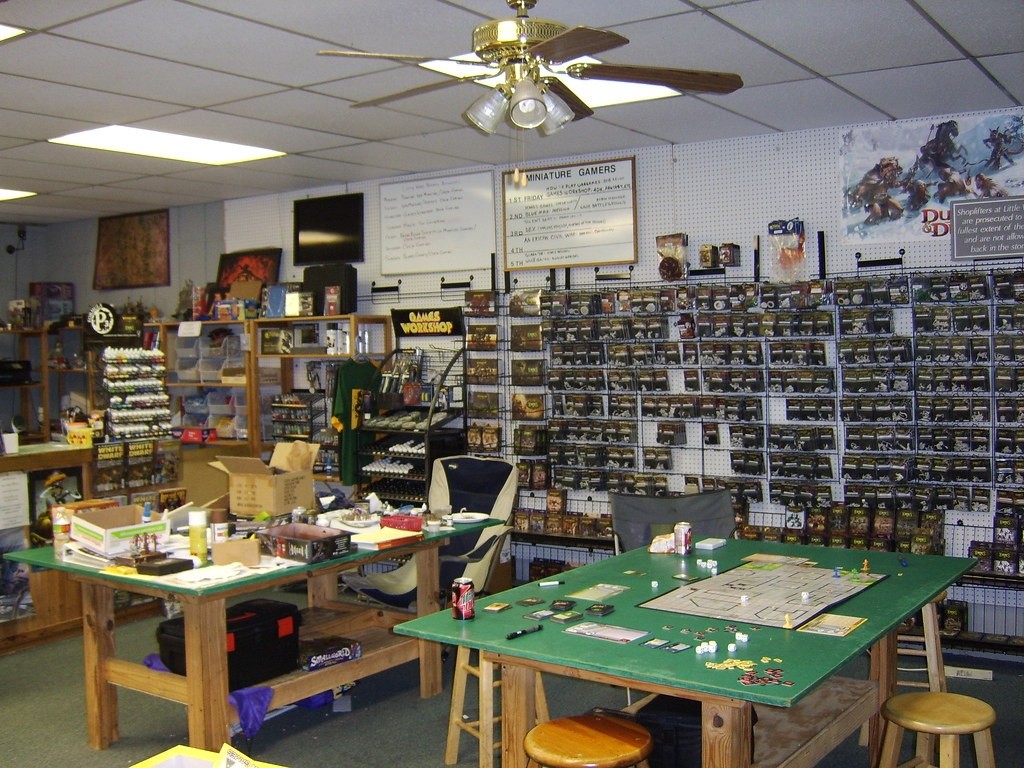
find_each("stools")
[878,688,999,768]
[858,591,949,748]
[523,713,656,768]
[446,649,549,767]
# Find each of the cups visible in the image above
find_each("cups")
[2,434,18,454]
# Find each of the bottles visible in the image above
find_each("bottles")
[189,512,207,565]
[211,508,229,542]
[53,507,71,558]
[316,515,329,527]
[327,322,369,355]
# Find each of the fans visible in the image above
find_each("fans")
[316,0,745,121]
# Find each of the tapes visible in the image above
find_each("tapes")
[252,511,271,522]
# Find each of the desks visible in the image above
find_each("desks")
[390,537,981,768]
[1,516,505,752]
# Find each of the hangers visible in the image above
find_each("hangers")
[350,344,369,362]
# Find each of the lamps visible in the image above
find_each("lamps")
[459,79,575,189]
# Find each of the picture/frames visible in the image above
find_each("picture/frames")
[500,156,640,268]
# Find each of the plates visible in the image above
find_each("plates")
[451,512,489,523]
[342,520,377,527]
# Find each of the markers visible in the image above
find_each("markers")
[538,581,565,586]
[142,502,152,523]
[897,555,908,567]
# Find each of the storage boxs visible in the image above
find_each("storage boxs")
[170,333,246,437]
[154,598,303,690]
[251,519,359,564]
[48,497,172,558]
[203,442,321,517]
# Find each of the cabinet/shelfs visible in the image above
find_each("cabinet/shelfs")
[270,386,326,444]
[144,321,252,458]
[0,319,100,445]
[249,312,393,481]
[358,343,469,506]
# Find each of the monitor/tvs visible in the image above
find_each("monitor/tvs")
[293,193,365,266]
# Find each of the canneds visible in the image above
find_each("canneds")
[451,578,475,621]
[674,521,692,556]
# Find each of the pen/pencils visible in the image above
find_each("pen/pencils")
[507,624,543,640]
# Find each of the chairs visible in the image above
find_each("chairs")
[344,455,520,660]
[608,489,741,704]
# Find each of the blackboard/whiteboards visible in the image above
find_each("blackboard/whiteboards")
[502,156,638,271]
[951,196,1024,259]
[379,168,495,275]
